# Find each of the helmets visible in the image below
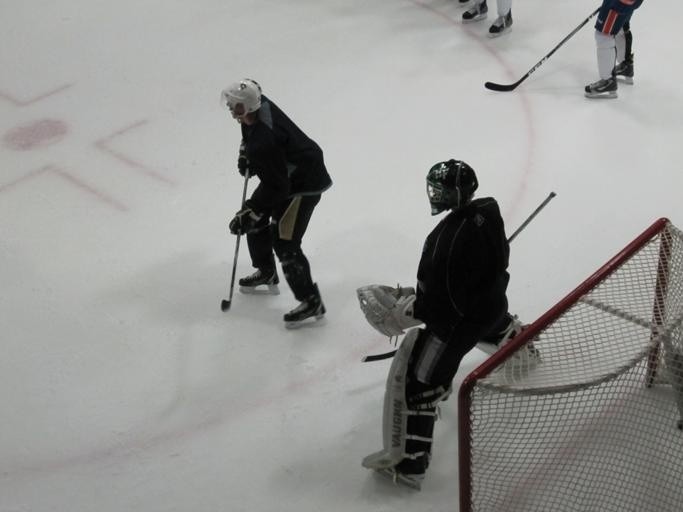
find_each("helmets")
[425,158,480,210]
[222,77,263,120]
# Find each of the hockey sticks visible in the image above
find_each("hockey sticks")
[360,193,556,362]
[485,6,601,91]
[222,160,250,312]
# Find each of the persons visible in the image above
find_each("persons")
[354,157,542,477]
[582,0,645,94]
[218,77,333,323]
[460,0,513,34]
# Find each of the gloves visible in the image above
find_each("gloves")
[228,197,273,236]
[236,137,257,180]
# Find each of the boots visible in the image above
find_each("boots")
[486,7,514,34]
[584,65,620,94]
[613,52,635,78]
[282,282,328,322]
[237,252,280,287]
[371,450,430,482]
[461,0,489,20]
